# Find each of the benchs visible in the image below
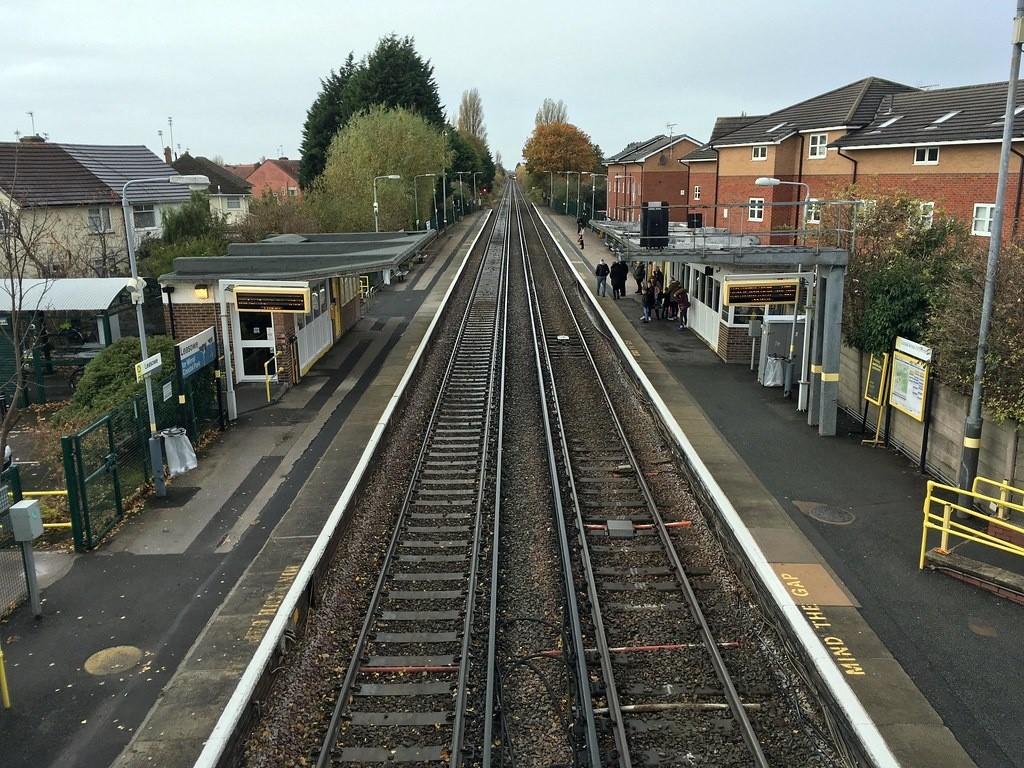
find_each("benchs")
[417,254,427,264]
[396,270,409,282]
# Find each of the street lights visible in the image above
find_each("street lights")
[473,172,483,211]
[567,171,580,220]
[122,174,211,498]
[459,171,472,217]
[755,176,810,400]
[543,170,553,207]
[373,175,401,291]
[450,172,463,225]
[581,171,595,232]
[589,174,609,246]
[442,130,448,236]
[414,174,436,231]
[614,175,636,222]
[557,171,569,215]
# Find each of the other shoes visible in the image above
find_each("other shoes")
[676,327,684,331]
[648,317,652,322]
[668,317,675,321]
[675,316,678,320]
[639,316,645,320]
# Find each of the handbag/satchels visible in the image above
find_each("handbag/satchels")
[681,302,691,310]
[576,239,581,244]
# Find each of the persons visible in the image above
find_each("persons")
[577,217,585,250]
[595,259,610,297]
[653,266,664,287]
[639,276,663,321]
[610,261,628,300]
[633,263,645,295]
[663,277,691,330]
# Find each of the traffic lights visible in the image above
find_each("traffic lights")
[483,186,487,193]
